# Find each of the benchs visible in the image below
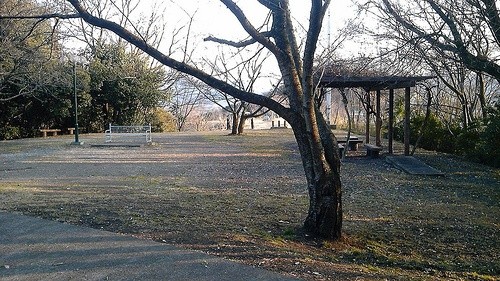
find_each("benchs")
[364,143,382,158]
[105,122,151,144]
[39,127,61,137]
[68,126,88,134]
[337,142,344,159]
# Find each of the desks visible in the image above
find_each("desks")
[348,138,363,151]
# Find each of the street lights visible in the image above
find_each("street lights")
[61,55,88,143]
